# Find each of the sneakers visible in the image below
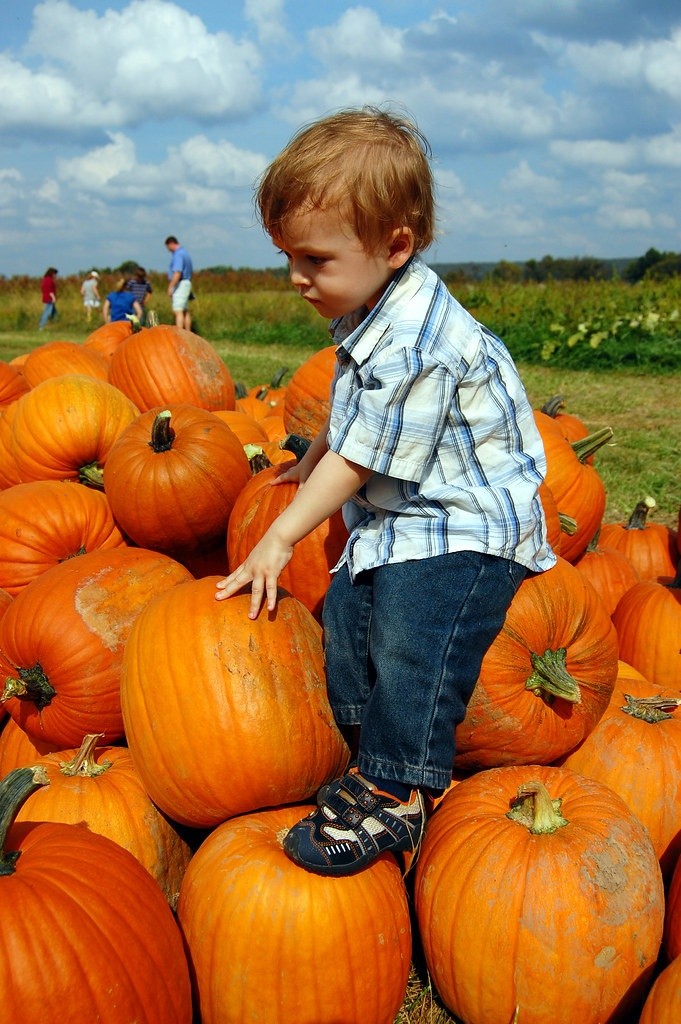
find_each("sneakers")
[282,770,432,876]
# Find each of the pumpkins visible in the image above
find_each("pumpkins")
[0,309,681,1024]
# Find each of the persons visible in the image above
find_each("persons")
[165,236,193,331]
[216,107,555,876]
[81,271,103,323]
[103,267,153,326]
[38,268,58,331]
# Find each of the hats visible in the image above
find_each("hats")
[90,271,100,280]
